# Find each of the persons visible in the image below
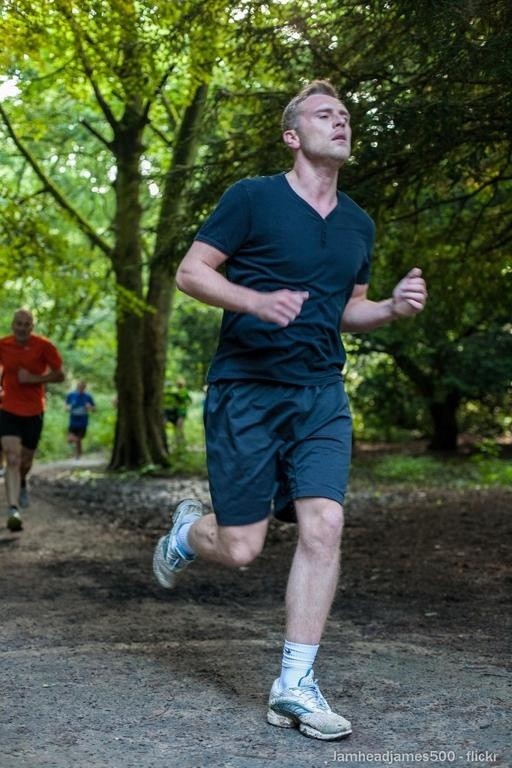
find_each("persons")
[64,379,97,457]
[170,376,193,449]
[152,78,429,741]
[0,311,65,532]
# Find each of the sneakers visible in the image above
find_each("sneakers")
[7,509,24,532]
[151,498,203,589]
[266,668,353,741]
